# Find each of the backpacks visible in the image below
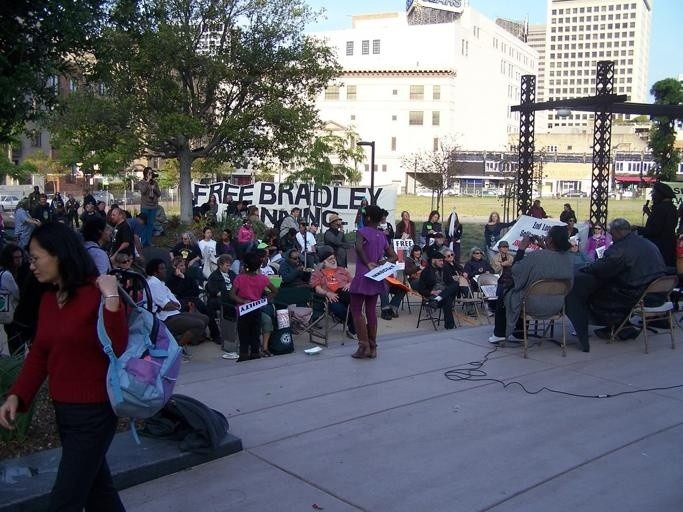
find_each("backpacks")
[273,214,294,237]
[97,284,184,445]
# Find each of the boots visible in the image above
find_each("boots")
[366,323,377,357]
[350,316,371,358]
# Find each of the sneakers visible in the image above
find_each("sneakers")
[213,334,274,363]
[487,332,505,343]
[507,335,525,342]
[181,357,190,364]
[180,346,193,359]
[390,309,399,317]
[428,302,495,331]
[380,308,392,320]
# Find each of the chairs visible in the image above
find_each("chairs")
[519,279,571,359]
[217,302,240,353]
[608,275,678,354]
[453,276,482,327]
[415,298,445,332]
[399,270,413,314]
[477,273,500,326]
[306,291,356,350]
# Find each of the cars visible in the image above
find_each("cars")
[415,189,436,197]
[561,189,587,197]
[47,194,68,208]
[443,190,458,197]
[0,194,19,212]
[478,190,497,197]
[83,191,114,206]
[116,191,141,204]
[589,190,616,199]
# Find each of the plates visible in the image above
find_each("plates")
[222,354,240,359]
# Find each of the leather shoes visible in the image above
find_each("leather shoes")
[618,327,640,340]
[593,327,615,339]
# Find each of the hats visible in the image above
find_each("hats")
[136,212,148,225]
[257,243,269,249]
[319,251,337,262]
[432,252,446,258]
[497,241,509,247]
[298,214,342,225]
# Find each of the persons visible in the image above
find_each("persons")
[642,184,679,317]
[579,218,669,339]
[1,185,613,360]
[0,220,132,511]
[133,166,161,226]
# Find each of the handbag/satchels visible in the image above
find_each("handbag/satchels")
[268,322,294,355]
[0,289,17,323]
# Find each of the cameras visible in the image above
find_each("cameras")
[149,171,159,182]
[528,235,538,243]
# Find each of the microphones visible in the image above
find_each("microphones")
[643,199,649,216]
[337,222,348,225]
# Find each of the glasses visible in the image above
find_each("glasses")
[473,253,483,254]
[448,254,453,257]
[329,257,336,262]
[290,256,299,260]
[120,259,130,264]
[29,254,49,263]
[611,231,616,236]
